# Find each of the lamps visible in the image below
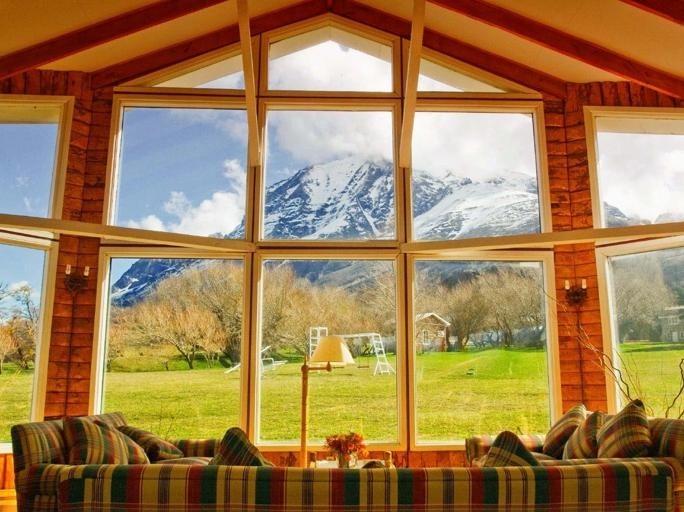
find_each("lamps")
[63,262,94,295]
[559,273,592,306]
[299,332,358,469]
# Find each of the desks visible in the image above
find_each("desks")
[305,457,398,471]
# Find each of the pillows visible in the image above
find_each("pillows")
[118,422,184,461]
[593,397,652,461]
[542,401,587,458]
[561,408,606,462]
[480,431,547,469]
[207,426,279,467]
[93,418,151,466]
[61,415,130,467]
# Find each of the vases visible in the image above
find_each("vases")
[336,451,358,470]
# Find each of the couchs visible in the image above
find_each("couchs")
[466,408,684,512]
[58,461,679,512]
[11,411,223,512]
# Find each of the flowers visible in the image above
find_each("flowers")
[320,430,371,463]
